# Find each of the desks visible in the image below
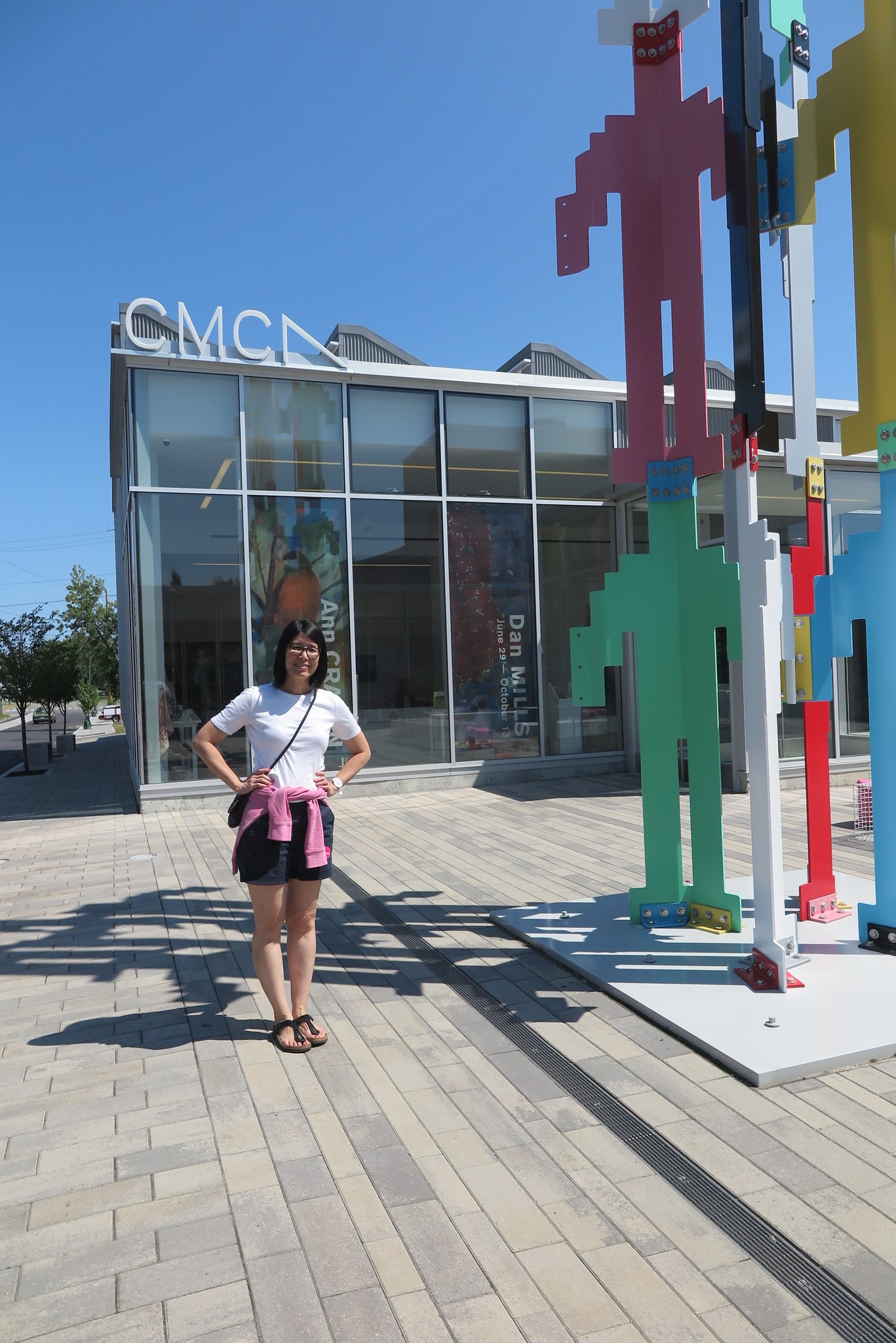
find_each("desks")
[170,709,201,780]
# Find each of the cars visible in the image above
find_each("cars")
[33,707,57,724]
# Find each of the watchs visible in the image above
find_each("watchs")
[331,777,343,795]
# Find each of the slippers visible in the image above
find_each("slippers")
[273,1014,311,1052]
[295,1014,328,1044]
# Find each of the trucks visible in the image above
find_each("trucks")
[103,705,122,722]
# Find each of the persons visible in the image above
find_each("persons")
[192,620,370,1052]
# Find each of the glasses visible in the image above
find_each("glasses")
[285,644,321,659]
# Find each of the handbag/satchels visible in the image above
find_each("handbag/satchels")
[228,789,252,828]
